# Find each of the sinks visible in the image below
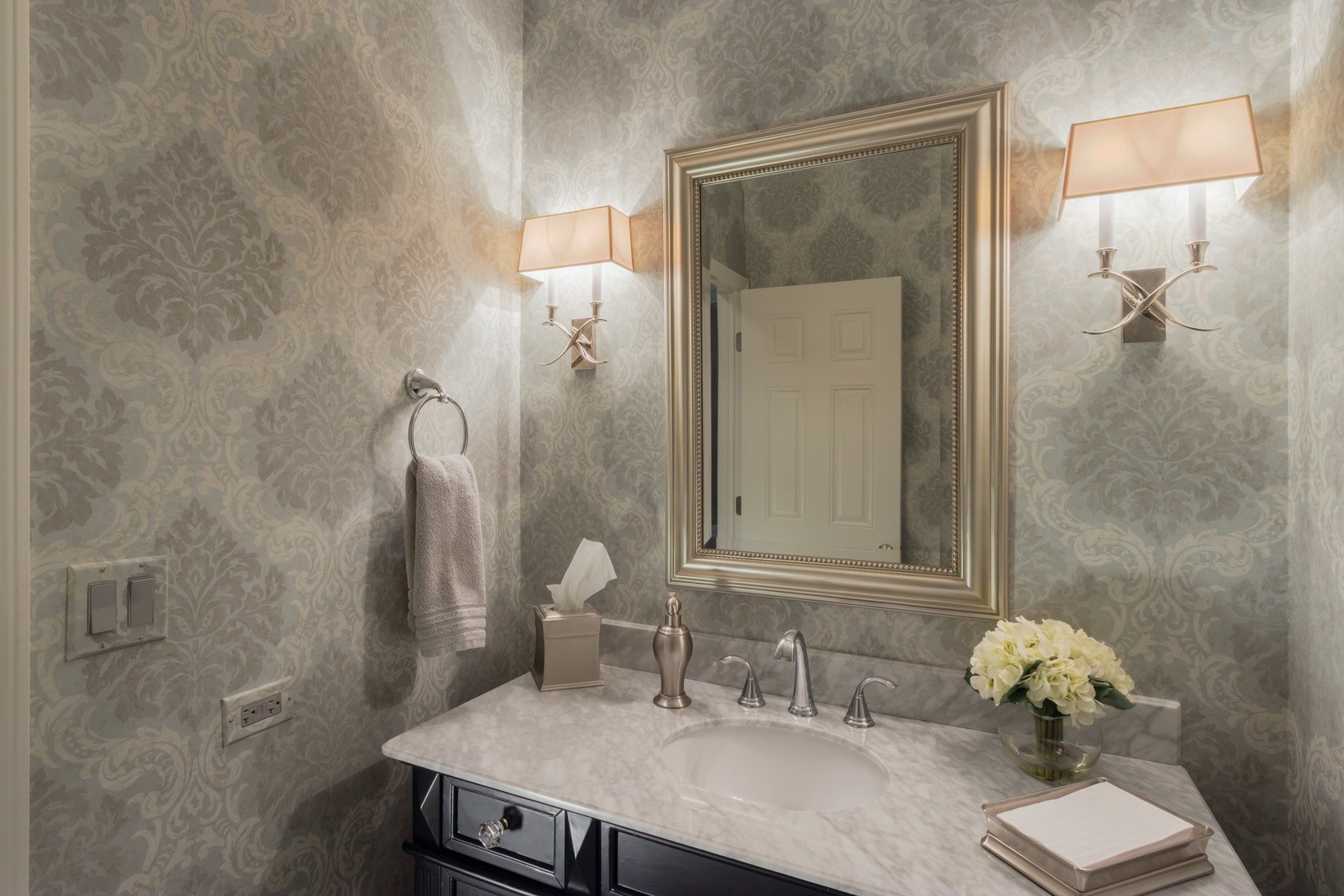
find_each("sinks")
[656,717,892,818]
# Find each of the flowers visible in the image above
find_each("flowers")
[964,616,1134,727]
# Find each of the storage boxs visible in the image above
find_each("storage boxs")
[982,775,1216,896]
[530,602,602,691]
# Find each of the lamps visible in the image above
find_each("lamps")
[1055,96,1266,344]
[518,203,636,373]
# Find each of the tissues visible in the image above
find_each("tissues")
[530,537,617,692]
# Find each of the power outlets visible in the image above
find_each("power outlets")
[222,679,294,747]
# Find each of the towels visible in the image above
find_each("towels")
[405,454,487,656]
[993,781,1194,867]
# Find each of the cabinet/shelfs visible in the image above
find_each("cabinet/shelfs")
[399,769,859,896]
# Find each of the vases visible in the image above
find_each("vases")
[998,699,1105,784]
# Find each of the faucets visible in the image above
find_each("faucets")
[773,629,818,717]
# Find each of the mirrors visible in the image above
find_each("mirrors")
[667,80,1008,620]
